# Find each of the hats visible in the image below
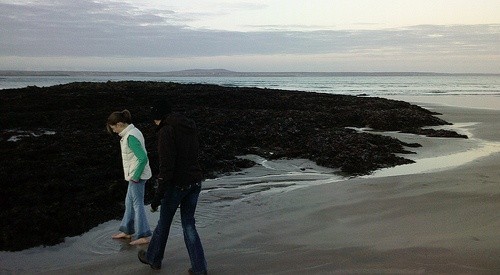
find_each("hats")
[150,104,171,120]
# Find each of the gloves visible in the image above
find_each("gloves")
[151,198,162,211]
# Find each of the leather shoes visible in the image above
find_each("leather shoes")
[188,268,208,275]
[138,249,161,270]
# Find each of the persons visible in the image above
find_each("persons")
[137,105,210,275]
[106,110,154,246]
